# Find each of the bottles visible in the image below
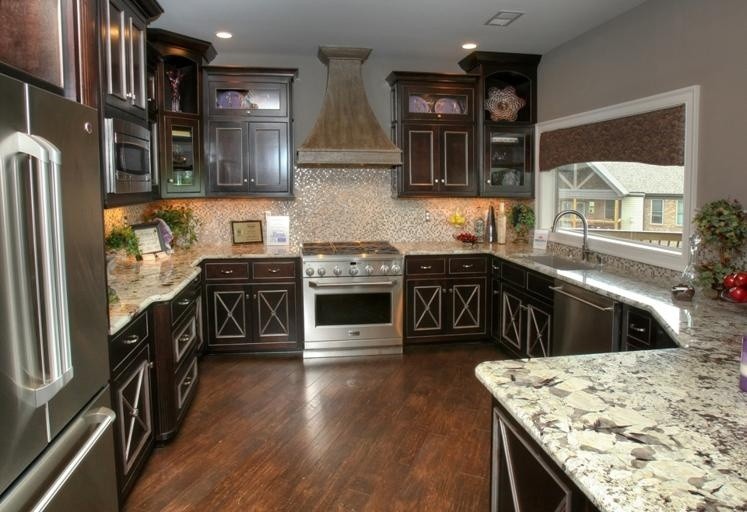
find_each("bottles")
[498,203,507,244]
[533,229,549,249]
[487,205,495,243]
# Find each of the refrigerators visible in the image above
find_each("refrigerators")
[1,74,126,512]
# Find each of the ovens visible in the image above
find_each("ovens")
[105,119,152,193]
[303,262,402,347]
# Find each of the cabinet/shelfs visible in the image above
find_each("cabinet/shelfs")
[402,254,490,347]
[385,71,478,198]
[490,252,557,360]
[620,307,678,353]
[0,0,80,102]
[80,0,152,132]
[479,65,535,197]
[154,44,207,198]
[110,301,155,512]
[490,388,601,512]
[204,258,304,350]
[156,260,205,446]
[205,64,299,198]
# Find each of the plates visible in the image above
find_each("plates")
[409,95,431,114]
[435,98,461,114]
[218,91,246,110]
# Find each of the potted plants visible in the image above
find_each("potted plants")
[685,200,747,302]
[509,203,534,243]
[148,205,199,248]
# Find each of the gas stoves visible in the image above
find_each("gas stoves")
[301,240,398,256]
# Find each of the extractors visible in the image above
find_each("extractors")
[297,46,401,169]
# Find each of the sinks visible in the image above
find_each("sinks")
[517,254,599,271]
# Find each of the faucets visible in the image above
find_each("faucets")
[552,209,590,268]
